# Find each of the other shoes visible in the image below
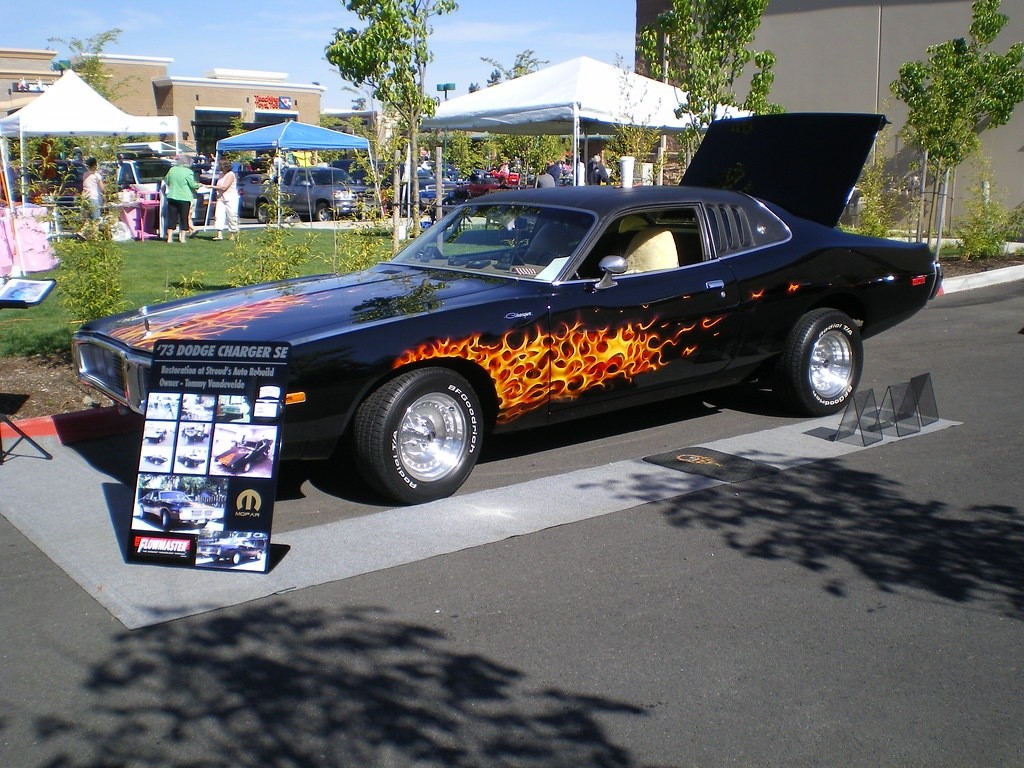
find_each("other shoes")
[212,236,223,241]
[226,236,239,241]
[189,229,200,239]
[182,241,188,244]
[76,233,87,241]
[167,241,173,243]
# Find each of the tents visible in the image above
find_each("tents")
[203,120,387,232]
[0,69,178,216]
[408,56,755,239]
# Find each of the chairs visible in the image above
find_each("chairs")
[595,227,678,278]
[521,223,583,266]
[0,393,54,465]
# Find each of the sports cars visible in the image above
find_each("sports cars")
[70,112,945,507]
[137,395,278,566]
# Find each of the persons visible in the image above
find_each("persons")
[160,152,239,243]
[76,158,107,240]
[535,153,612,189]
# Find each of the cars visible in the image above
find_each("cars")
[5,154,530,223]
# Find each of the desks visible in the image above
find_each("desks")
[50,201,142,244]
